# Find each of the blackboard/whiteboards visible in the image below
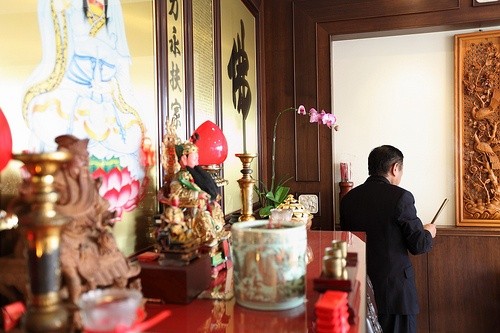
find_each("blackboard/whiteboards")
[329,22,500,227]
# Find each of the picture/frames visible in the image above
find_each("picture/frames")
[454,30,500,228]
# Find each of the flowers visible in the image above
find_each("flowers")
[257,104,339,219]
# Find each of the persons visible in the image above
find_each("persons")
[158,141,231,248]
[339,145,436,333]
[15,135,142,308]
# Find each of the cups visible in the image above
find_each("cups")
[75,288,141,333]
[324,247,343,263]
[232,221,307,311]
[332,239,349,262]
[323,255,342,280]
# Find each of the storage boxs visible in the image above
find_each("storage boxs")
[139,256,212,304]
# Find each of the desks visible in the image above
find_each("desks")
[141,231,367,333]
